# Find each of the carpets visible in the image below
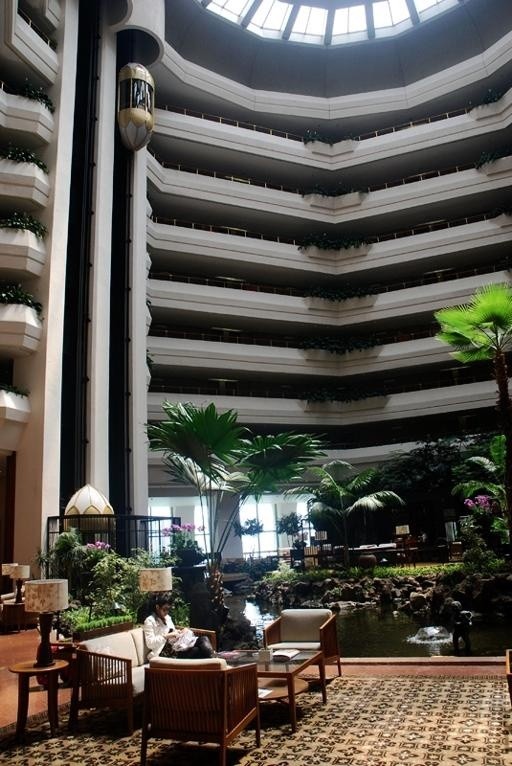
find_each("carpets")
[0,672,511,766]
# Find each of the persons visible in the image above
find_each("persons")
[144,594,216,658]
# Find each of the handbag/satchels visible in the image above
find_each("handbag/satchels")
[170,628,198,651]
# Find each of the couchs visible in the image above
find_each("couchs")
[0,591,39,630]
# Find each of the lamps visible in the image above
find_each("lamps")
[0,563,17,575]
[19,578,71,665]
[138,567,173,607]
[10,565,29,602]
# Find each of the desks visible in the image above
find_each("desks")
[7,659,82,736]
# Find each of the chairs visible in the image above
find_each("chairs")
[262,607,342,703]
[66,621,222,736]
[139,655,261,765]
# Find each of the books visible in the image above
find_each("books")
[273,648,300,660]
